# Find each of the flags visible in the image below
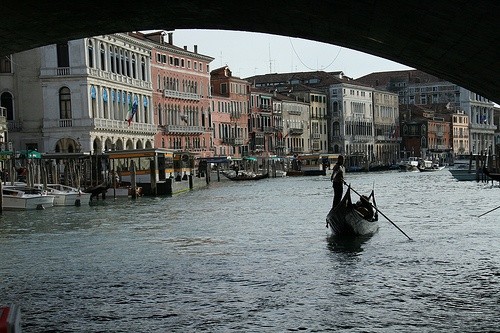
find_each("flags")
[125,102,137,126]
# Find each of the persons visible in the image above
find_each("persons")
[331,155,345,208]
[115,166,123,184]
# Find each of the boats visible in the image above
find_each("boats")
[399,148,500,183]
[0,164,92,211]
[326,181,380,240]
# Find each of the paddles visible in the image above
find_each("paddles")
[477,205,500,218]
[344,181,413,240]
